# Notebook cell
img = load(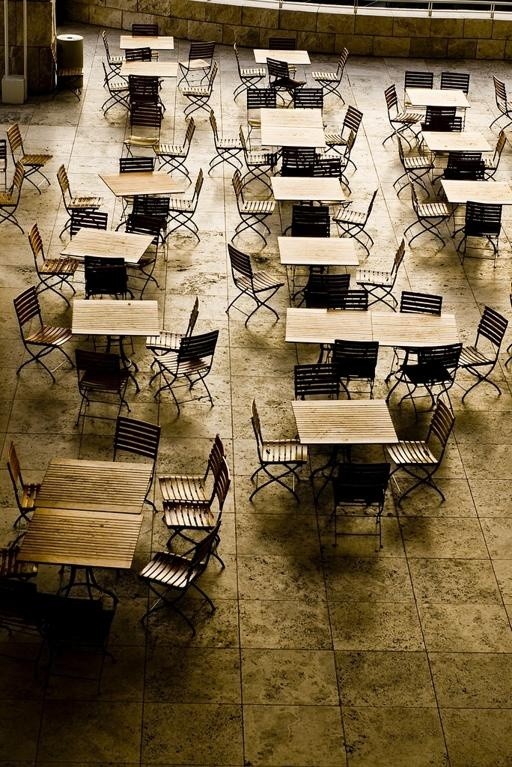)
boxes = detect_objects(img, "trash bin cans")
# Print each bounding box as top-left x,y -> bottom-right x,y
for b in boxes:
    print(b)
56,34 -> 83,89
